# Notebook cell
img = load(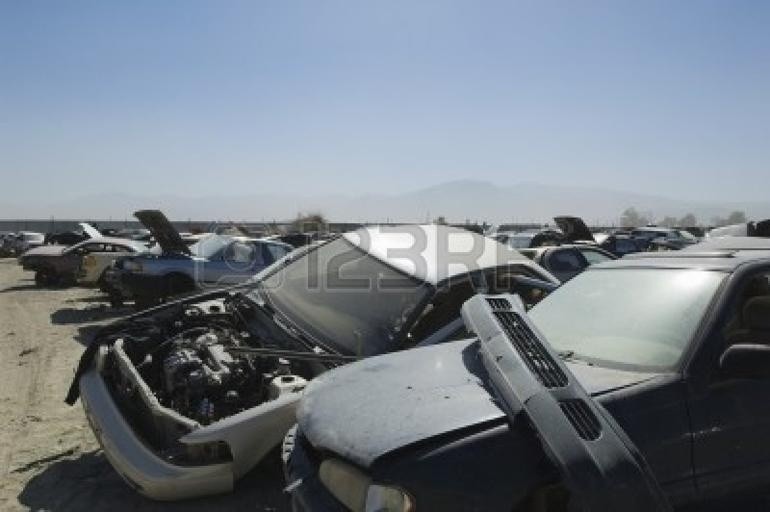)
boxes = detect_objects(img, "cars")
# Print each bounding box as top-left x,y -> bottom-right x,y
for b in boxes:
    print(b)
75,221 -> 561,500
281,243 -> 769,508
